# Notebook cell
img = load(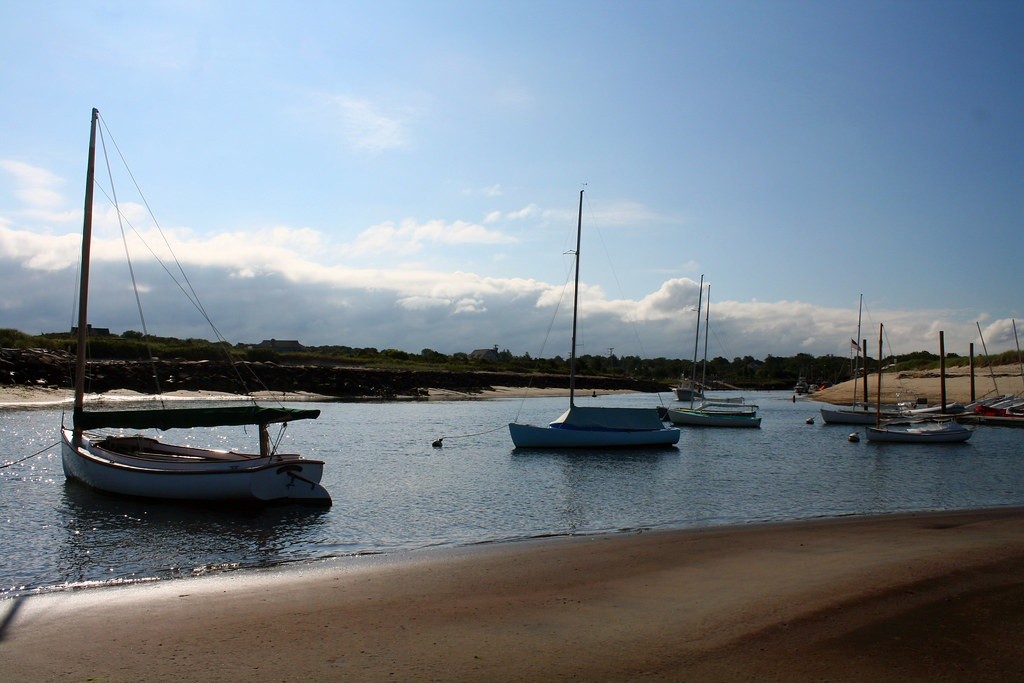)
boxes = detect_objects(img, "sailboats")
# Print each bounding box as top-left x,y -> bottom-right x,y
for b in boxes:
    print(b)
508,181 -> 682,451
655,272 -> 763,430
794,294 -> 1024,445
56,104 -> 333,512
